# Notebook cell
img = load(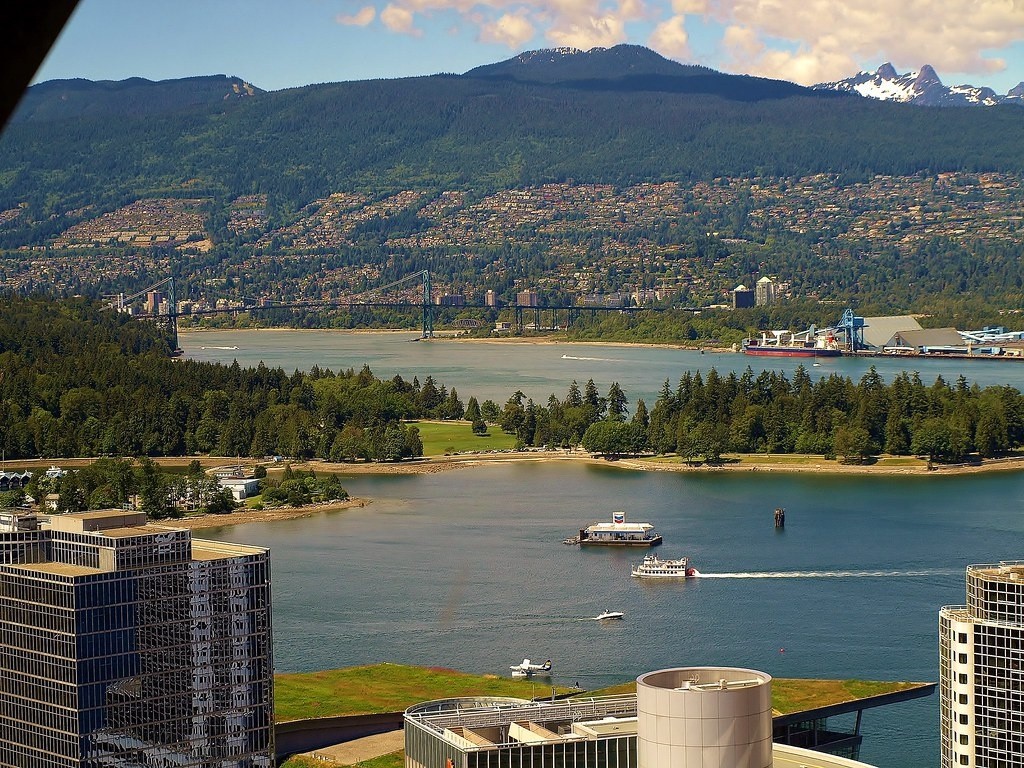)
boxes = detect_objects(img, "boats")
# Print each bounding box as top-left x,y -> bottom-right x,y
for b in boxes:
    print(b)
509,658 -> 552,678
629,552 -> 688,577
597,610 -> 624,619
